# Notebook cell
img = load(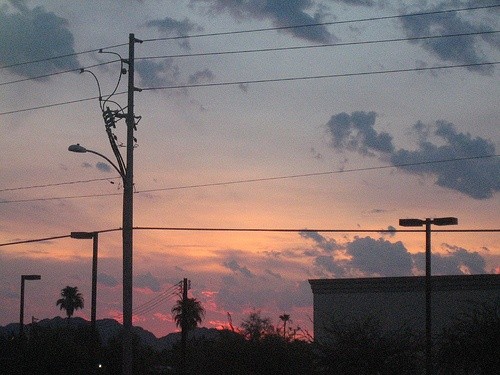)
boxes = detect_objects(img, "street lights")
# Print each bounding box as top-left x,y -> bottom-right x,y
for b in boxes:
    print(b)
19,275 -> 41,335
399,215 -> 457,375
69,231 -> 98,330
67,144 -> 131,375
